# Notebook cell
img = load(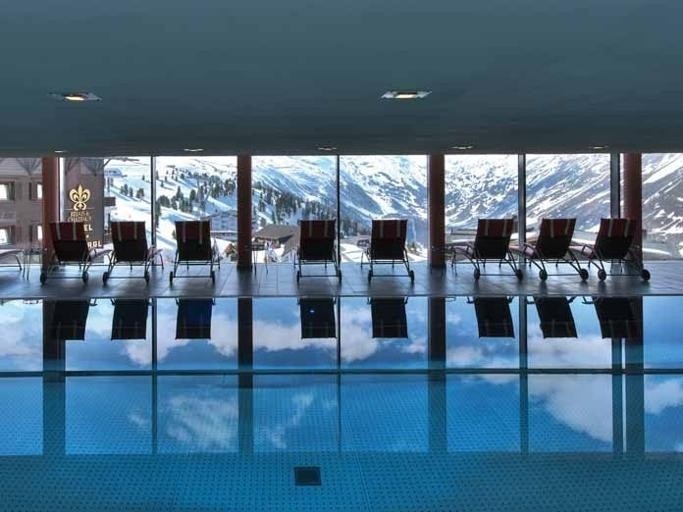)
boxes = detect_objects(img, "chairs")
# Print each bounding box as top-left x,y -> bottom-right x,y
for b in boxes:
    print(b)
293,218 -> 342,284
360,218 -> 414,286
103,221 -> 164,286
499,217 -> 589,281
40,221 -> 113,285
0,248 -> 25,271
169,218 -> 220,286
556,217 -> 651,281
451,218 -> 524,283
